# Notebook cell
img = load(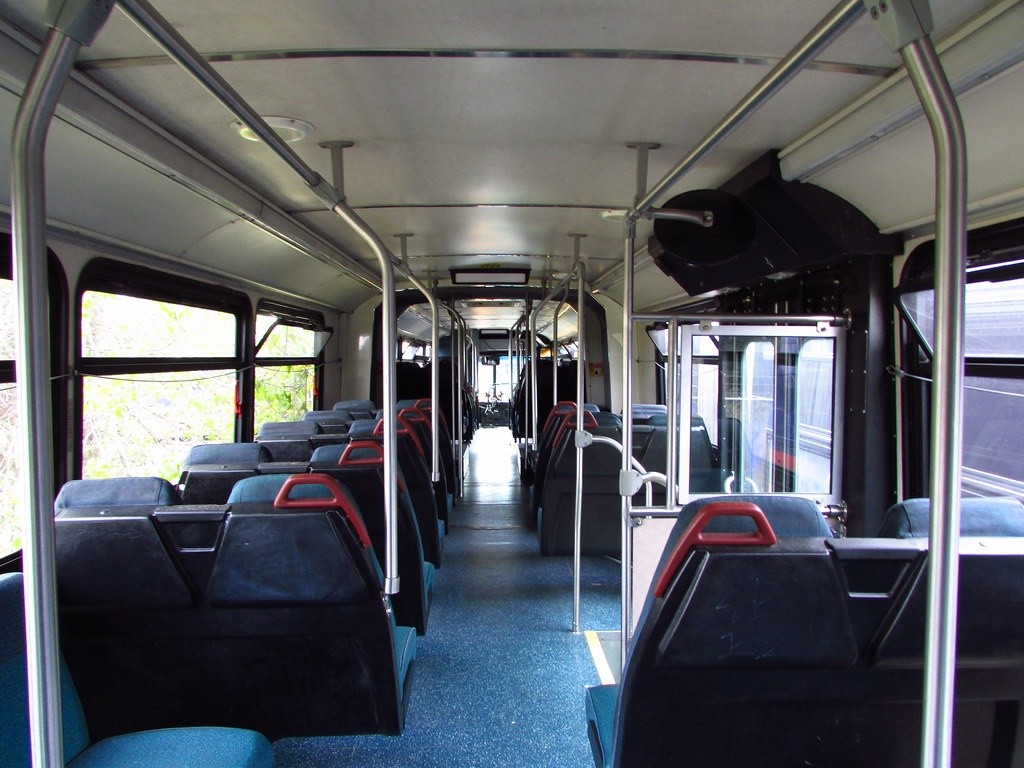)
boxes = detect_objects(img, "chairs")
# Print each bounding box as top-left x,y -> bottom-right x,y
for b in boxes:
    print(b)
0,389 -> 1024,768
